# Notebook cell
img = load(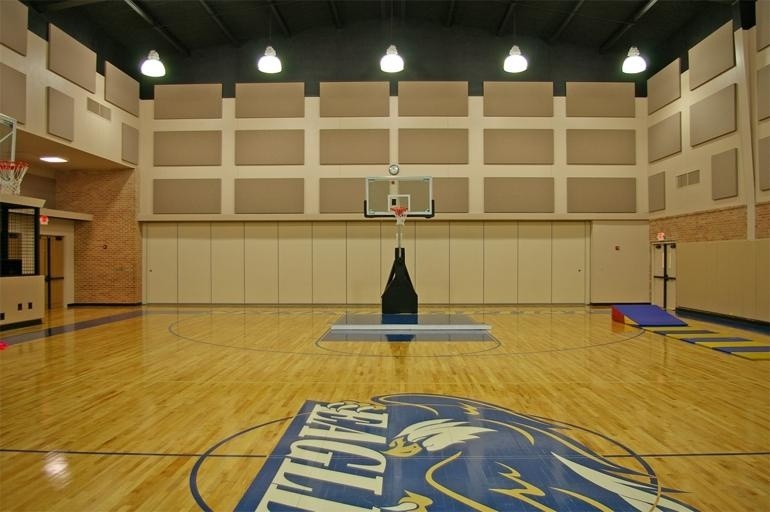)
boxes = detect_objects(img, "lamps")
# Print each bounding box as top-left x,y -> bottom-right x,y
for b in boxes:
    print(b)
141,0 -> 165,78
503,0 -> 528,73
257,0 -> 283,74
380,0 -> 404,74
620,0 -> 648,75
